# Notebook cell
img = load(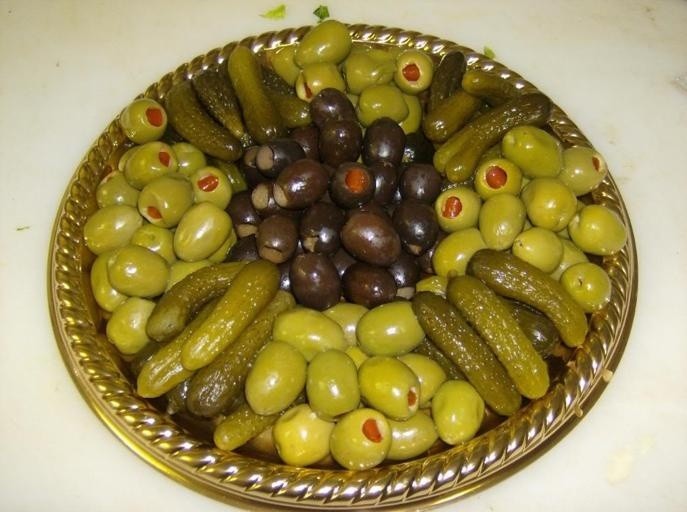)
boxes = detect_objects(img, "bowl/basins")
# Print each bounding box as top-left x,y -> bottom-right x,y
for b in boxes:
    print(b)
46,18 -> 640,512
